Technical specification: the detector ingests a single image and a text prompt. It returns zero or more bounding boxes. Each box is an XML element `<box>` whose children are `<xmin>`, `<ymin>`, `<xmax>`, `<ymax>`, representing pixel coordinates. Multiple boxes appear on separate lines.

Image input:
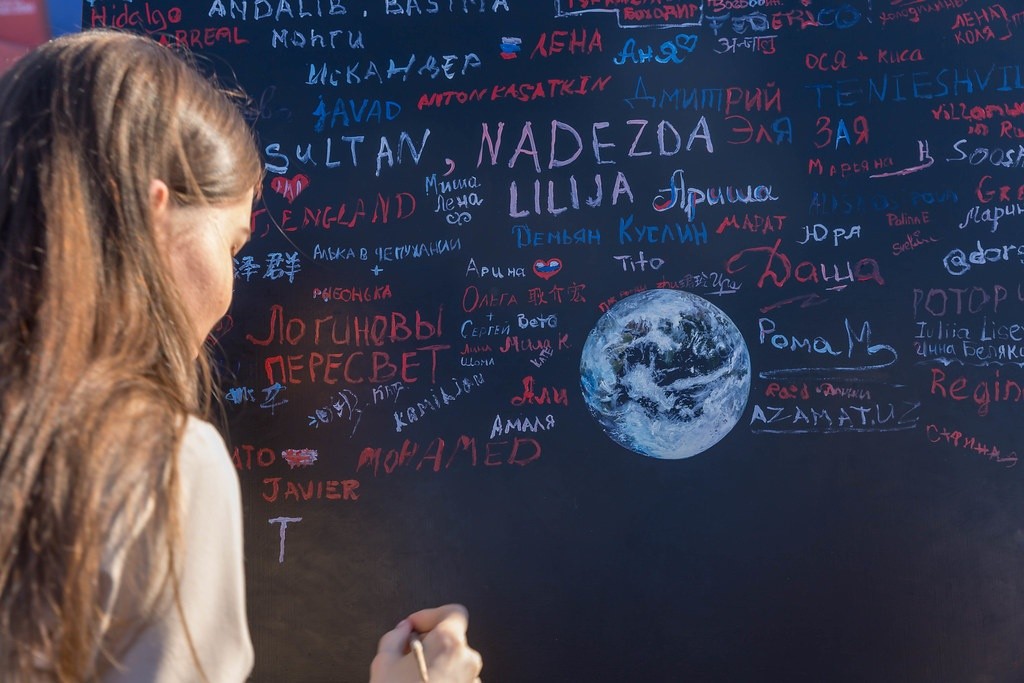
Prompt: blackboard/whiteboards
<box><xmin>82</xmin><ymin>0</ymin><xmax>1024</xmax><ymax>683</ymax></box>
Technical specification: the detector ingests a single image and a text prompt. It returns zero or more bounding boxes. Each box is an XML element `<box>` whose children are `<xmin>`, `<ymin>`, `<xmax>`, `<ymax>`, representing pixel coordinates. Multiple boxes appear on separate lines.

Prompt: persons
<box><xmin>0</xmin><ymin>30</ymin><xmax>483</xmax><ymax>683</ymax></box>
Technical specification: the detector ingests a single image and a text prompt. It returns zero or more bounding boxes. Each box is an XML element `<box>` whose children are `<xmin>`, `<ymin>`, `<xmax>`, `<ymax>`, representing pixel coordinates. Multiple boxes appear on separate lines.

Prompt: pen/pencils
<box><xmin>408</xmin><ymin>632</ymin><xmax>429</xmax><ymax>682</ymax></box>
